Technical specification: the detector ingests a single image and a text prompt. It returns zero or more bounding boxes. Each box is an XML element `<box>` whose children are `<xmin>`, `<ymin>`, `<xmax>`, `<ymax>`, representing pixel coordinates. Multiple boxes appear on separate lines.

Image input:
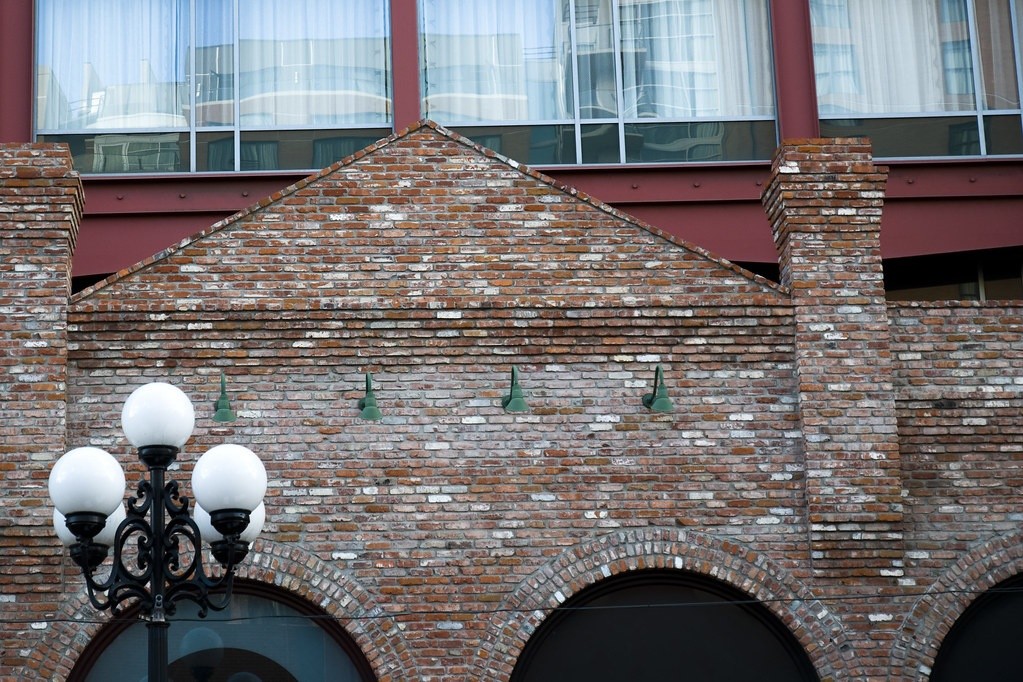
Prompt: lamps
<box><xmin>357</xmin><ymin>372</ymin><xmax>383</xmax><ymax>420</ymax></box>
<box><xmin>501</xmin><ymin>365</ymin><xmax>529</xmax><ymax>414</ymax></box>
<box><xmin>642</xmin><ymin>366</ymin><xmax>675</xmax><ymax>412</ymax></box>
<box><xmin>211</xmin><ymin>373</ymin><xmax>236</xmax><ymax>424</ymax></box>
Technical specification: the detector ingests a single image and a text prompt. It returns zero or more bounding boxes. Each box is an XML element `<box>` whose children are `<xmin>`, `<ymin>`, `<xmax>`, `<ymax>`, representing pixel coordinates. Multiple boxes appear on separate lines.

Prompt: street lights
<box><xmin>43</xmin><ymin>382</ymin><xmax>271</xmax><ymax>682</ymax></box>
